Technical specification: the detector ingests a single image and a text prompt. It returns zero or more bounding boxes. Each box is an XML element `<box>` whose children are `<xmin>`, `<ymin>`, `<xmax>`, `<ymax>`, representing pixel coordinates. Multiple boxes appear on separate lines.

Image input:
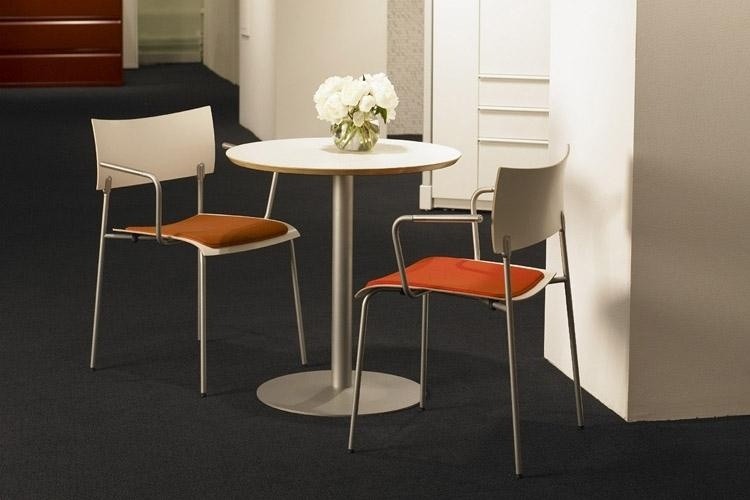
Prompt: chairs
<box><xmin>90</xmin><ymin>105</ymin><xmax>308</xmax><ymax>398</ymax></box>
<box><xmin>348</xmin><ymin>143</ymin><xmax>584</xmax><ymax>477</ymax></box>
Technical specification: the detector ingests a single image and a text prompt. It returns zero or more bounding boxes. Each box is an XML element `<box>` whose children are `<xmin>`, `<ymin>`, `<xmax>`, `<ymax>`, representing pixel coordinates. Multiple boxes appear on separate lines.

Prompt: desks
<box><xmin>226</xmin><ymin>136</ymin><xmax>464</xmax><ymax>418</ymax></box>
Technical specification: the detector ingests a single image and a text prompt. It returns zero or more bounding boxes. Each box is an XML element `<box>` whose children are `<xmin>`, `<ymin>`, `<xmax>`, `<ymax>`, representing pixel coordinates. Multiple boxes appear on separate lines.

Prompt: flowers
<box><xmin>312</xmin><ymin>71</ymin><xmax>401</xmax><ymax>152</ymax></box>
